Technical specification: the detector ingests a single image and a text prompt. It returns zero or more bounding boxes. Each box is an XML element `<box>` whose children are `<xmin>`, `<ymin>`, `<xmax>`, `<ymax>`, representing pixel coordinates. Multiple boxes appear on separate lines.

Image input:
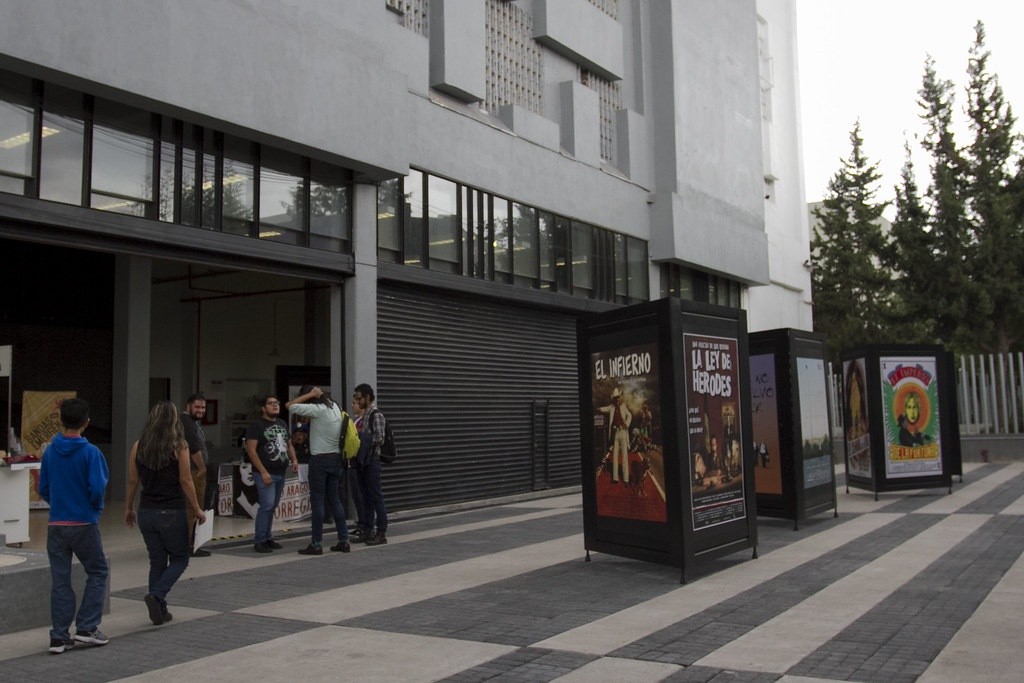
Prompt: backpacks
<box><xmin>368</xmin><ymin>409</ymin><xmax>396</xmax><ymax>466</ymax></box>
<box><xmin>329</xmin><ymin>400</ymin><xmax>361</xmax><ymax>459</ymax></box>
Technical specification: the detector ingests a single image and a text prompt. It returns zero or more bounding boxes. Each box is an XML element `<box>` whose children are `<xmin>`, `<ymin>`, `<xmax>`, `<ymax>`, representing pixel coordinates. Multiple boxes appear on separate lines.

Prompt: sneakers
<box><xmin>49</xmin><ymin>638</ymin><xmax>75</xmax><ymax>653</ymax></box>
<box><xmin>74</xmin><ymin>627</ymin><xmax>109</xmax><ymax>644</ymax></box>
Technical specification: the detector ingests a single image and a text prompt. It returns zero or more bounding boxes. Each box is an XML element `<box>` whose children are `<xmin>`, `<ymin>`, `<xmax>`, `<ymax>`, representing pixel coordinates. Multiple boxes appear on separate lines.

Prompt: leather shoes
<box><xmin>330</xmin><ymin>542</ymin><xmax>350</xmax><ymax>552</ymax></box>
<box><xmin>297</xmin><ymin>544</ymin><xmax>323</xmax><ymax>555</ymax></box>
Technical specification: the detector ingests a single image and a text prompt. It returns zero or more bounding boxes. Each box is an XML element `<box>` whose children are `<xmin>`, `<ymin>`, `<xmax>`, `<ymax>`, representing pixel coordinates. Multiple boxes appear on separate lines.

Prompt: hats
<box><xmin>611</xmin><ymin>388</ymin><xmax>622</xmax><ymax>400</ymax></box>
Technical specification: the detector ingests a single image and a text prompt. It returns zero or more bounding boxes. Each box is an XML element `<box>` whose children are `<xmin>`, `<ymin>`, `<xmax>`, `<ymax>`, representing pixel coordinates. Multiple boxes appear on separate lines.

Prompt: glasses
<box><xmin>265</xmin><ymin>401</ymin><xmax>280</xmax><ymax>405</ymax></box>
<box><xmin>355</xmin><ymin>397</ymin><xmax>363</xmax><ymax>401</ymax></box>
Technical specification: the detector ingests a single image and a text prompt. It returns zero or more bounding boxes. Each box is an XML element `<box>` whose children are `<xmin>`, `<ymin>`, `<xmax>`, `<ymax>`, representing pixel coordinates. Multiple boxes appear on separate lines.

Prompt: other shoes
<box><xmin>190</xmin><ymin>548</ymin><xmax>211</xmax><ymax>557</ymax></box>
<box><xmin>267</xmin><ymin>540</ymin><xmax>282</xmax><ymax>549</ymax></box>
<box><xmin>152</xmin><ymin>612</ymin><xmax>173</xmax><ymax>625</ymax></box>
<box><xmin>350</xmin><ymin>527</ymin><xmax>374</xmax><ymax>543</ymax></box>
<box><xmin>255</xmin><ymin>543</ymin><xmax>273</xmax><ymax>553</ymax></box>
<box><xmin>366</xmin><ymin>528</ymin><xmax>387</xmax><ymax>545</ymax></box>
<box><xmin>144</xmin><ymin>595</ymin><xmax>161</xmax><ymax>622</ymax></box>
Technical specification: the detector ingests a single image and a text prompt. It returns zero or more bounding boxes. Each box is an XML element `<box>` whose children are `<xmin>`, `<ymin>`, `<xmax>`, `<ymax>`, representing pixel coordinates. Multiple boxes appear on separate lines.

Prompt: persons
<box><xmin>906</xmin><ymin>393</ymin><xmax>920</xmax><ymax>423</ymax></box>
<box><xmin>180</xmin><ymin>394</ymin><xmax>211</xmax><ymax>557</ymax></box>
<box><xmin>754</xmin><ymin>440</ymin><xmax>768</xmax><ymax>468</ymax></box>
<box><xmin>124</xmin><ymin>401</ymin><xmax>206</xmax><ymax>625</ymax></box>
<box><xmin>247</xmin><ymin>396</ymin><xmax>298</xmax><ymax>552</ymax></box>
<box><xmin>693</xmin><ymin>399</ymin><xmax>741</xmax><ymax>488</ymax></box>
<box><xmin>596</xmin><ymin>388</ymin><xmax>652</xmax><ymax>487</ymax></box>
<box><xmin>286</xmin><ymin>385</ymin><xmax>350</xmax><ymax>554</ymax></box>
<box><xmin>350</xmin><ymin>383</ymin><xmax>388</xmax><ymax>545</ymax></box>
<box><xmin>38</xmin><ymin>398</ymin><xmax>110</xmax><ymax>653</ymax></box>
